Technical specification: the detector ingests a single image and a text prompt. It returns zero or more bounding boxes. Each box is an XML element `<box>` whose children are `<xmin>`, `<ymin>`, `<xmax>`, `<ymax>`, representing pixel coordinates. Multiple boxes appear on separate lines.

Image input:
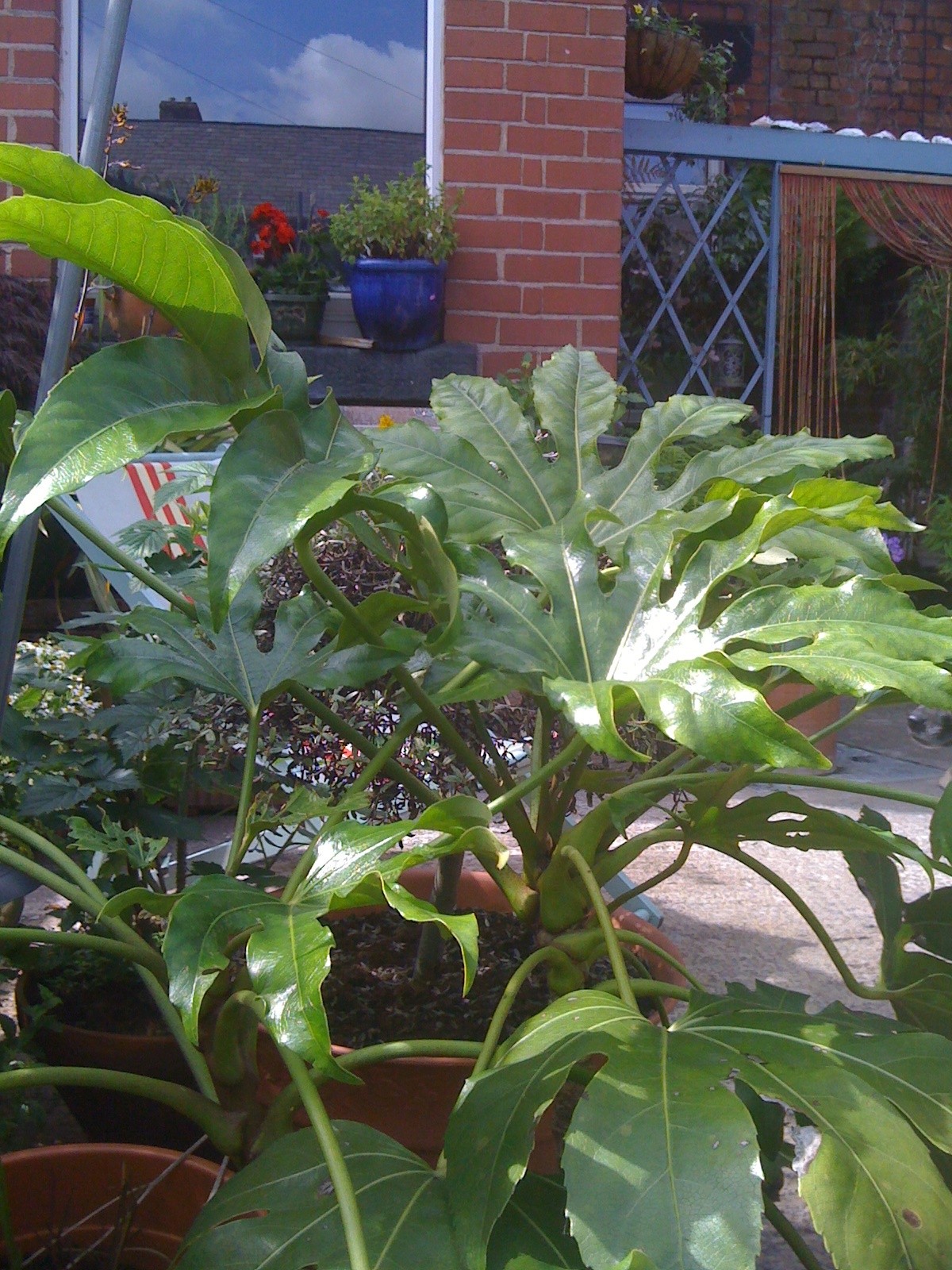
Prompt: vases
<box><xmin>627</xmin><ymin>22</ymin><xmax>700</xmax><ymax>96</ymax></box>
<box><xmin>262</xmin><ymin>295</ymin><xmax>335</xmax><ymax>345</ymax></box>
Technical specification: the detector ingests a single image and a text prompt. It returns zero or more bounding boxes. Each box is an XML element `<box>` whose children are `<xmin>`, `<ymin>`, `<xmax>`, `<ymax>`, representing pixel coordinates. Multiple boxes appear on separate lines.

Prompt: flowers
<box><xmin>244</xmin><ymin>201</ymin><xmax>330</xmax><ymax>292</ymax></box>
<box><xmin>625</xmin><ymin>2</ymin><xmax>746</xmax><ymax>123</ymax></box>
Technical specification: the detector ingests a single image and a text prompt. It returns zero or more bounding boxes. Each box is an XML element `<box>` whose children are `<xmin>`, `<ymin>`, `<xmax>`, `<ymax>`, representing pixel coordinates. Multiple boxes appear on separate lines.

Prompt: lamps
<box><xmin>709</xmin><ymin>331</ymin><xmax>746</xmax><ymax>398</ymax></box>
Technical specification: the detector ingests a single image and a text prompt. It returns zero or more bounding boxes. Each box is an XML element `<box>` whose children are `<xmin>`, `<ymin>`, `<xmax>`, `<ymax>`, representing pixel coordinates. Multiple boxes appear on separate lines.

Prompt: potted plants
<box><xmin>1</xmin><ymin>142</ymin><xmax>952</xmax><ymax>1269</ymax></box>
